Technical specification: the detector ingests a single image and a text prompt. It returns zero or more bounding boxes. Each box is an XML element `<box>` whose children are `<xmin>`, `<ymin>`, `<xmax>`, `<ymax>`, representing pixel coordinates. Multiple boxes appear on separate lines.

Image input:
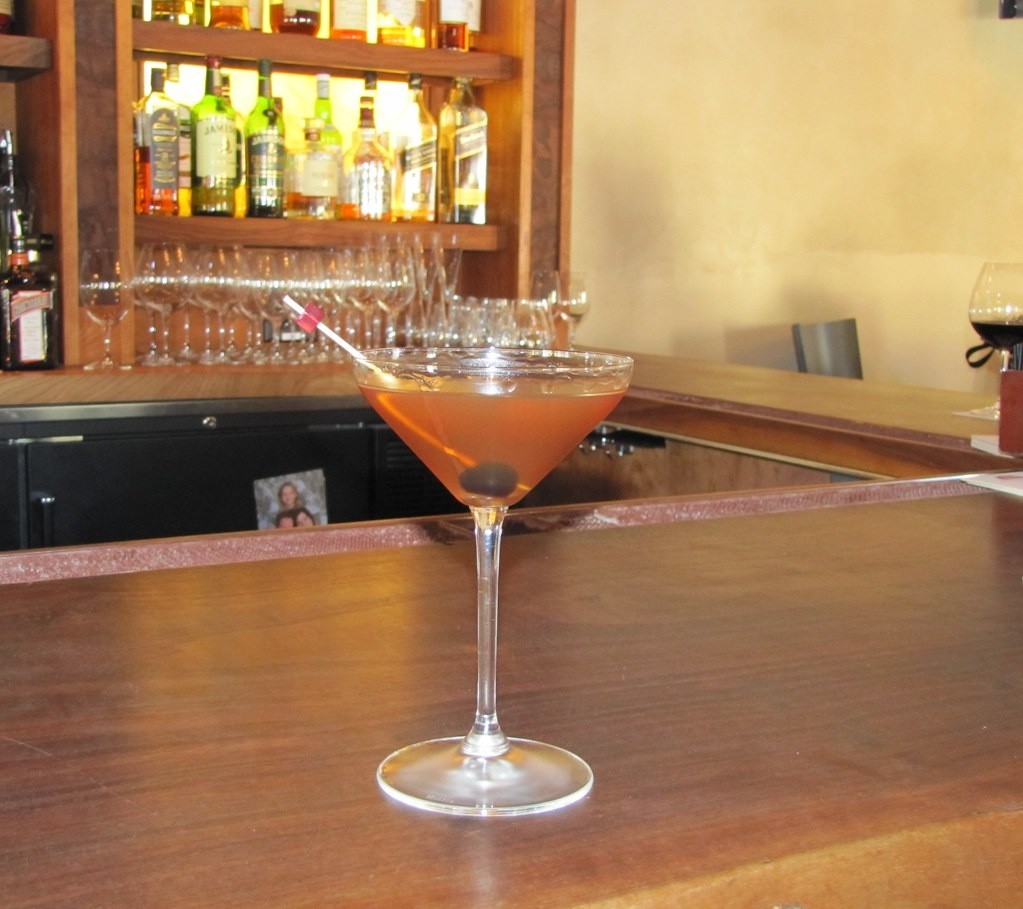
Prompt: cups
<box><xmin>967</xmin><ymin>260</ymin><xmax>1023</xmax><ymax>418</ymax></box>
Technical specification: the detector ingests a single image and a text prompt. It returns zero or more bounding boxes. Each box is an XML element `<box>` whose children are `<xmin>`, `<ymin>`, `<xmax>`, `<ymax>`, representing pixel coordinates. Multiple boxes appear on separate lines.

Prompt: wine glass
<box><xmin>76</xmin><ymin>233</ymin><xmax>591</xmax><ymax>369</ymax></box>
<box><xmin>351</xmin><ymin>347</ymin><xmax>634</xmax><ymax>815</ymax></box>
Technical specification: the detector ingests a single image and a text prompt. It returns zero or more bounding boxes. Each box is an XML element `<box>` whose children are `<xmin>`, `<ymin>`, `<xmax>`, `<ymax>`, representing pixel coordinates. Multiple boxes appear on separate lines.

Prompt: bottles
<box><xmin>132</xmin><ymin>0</ymin><xmax>489</xmax><ymax>225</ymax></box>
<box><xmin>0</xmin><ymin>129</ymin><xmax>61</xmax><ymax>368</ymax></box>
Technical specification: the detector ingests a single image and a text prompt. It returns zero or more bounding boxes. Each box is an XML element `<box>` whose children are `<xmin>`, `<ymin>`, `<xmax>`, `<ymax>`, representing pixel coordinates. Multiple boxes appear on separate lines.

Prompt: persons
<box><xmin>277</xmin><ymin>482</ymin><xmax>305</xmax><ymax>512</ymax></box>
<box><xmin>275</xmin><ymin>506</ymin><xmax>315</xmax><ymax>529</ymax></box>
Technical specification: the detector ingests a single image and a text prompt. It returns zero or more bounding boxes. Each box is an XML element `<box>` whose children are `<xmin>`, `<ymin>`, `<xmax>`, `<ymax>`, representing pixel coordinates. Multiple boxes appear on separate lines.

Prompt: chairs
<box><xmin>791</xmin><ymin>316</ymin><xmax>863</xmax><ymax>381</ymax></box>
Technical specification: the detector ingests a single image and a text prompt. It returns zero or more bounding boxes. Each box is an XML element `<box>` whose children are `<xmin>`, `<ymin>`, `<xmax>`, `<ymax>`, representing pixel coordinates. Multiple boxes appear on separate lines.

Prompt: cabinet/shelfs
<box><xmin>25</xmin><ymin>424</ymin><xmax>375</xmax><ymax>551</ymax></box>
<box><xmin>115</xmin><ymin>0</ymin><xmax>537</xmax><ymax>367</ymax></box>
<box><xmin>0</xmin><ymin>0</ymin><xmax>80</xmax><ymax>370</ymax></box>
